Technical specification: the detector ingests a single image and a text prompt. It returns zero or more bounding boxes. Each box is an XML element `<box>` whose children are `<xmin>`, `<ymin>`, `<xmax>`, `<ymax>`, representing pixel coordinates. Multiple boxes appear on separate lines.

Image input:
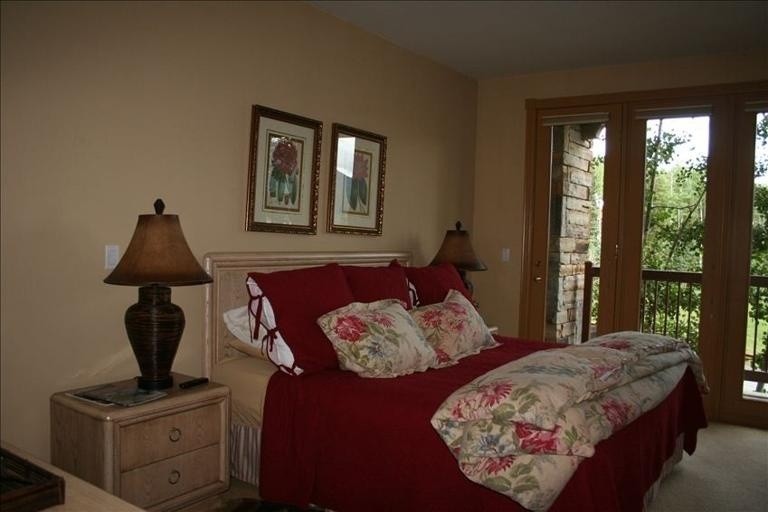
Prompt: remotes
<box><xmin>178</xmin><ymin>378</ymin><xmax>209</xmax><ymax>390</ymax></box>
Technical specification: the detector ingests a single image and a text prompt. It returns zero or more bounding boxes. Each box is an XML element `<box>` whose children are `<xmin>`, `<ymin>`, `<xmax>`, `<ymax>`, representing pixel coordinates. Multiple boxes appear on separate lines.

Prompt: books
<box><xmin>65</xmin><ymin>386</ymin><xmax>115</xmax><ymax>406</ymax></box>
<box><xmin>79</xmin><ymin>385</ymin><xmax>168</xmax><ymax>407</ymax></box>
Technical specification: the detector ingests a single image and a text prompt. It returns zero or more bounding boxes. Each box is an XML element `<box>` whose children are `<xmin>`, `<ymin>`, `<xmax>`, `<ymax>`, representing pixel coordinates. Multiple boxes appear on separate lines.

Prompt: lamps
<box><xmin>103</xmin><ymin>198</ymin><xmax>213</xmax><ymax>391</ymax></box>
<box><xmin>427</xmin><ymin>221</ymin><xmax>487</xmax><ymax>294</ymax></box>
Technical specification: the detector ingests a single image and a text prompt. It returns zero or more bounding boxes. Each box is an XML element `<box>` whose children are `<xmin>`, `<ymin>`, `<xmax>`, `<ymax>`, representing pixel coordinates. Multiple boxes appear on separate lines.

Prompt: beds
<box><xmin>203</xmin><ymin>250</ymin><xmax>710</xmax><ymax>512</ymax></box>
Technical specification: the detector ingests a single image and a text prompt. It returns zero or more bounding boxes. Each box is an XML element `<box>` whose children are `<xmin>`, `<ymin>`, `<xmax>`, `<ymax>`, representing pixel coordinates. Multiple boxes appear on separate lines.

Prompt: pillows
<box><xmin>341</xmin><ymin>259</ymin><xmax>412</xmax><ymax>311</ymax></box>
<box><xmin>316</xmin><ymin>298</ymin><xmax>439</xmax><ymax>379</ymax></box>
<box><xmin>228</xmin><ymin>339</ymin><xmax>263</xmax><ymax>357</ymax></box>
<box><xmin>245</xmin><ymin>259</ymin><xmax>356</xmax><ymax>377</ymax></box>
<box><xmin>403</xmin><ymin>252</ymin><xmax>478</xmax><ymax>311</ymax></box>
<box><xmin>408</xmin><ymin>289</ymin><xmax>504</xmax><ymax>368</ymax></box>
<box><xmin>223</xmin><ymin>303</ymin><xmax>267</xmax><ymax>348</ymax></box>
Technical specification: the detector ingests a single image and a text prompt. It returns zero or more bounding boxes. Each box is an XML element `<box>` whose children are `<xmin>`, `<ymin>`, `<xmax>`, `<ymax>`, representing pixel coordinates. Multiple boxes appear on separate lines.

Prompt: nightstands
<box><xmin>50</xmin><ymin>372</ymin><xmax>232</xmax><ymax>512</ymax></box>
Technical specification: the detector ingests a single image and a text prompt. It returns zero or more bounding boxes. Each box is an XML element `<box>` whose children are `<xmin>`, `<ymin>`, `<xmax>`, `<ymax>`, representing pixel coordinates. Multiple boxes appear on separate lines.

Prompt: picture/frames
<box><xmin>244</xmin><ymin>104</ymin><xmax>323</xmax><ymax>236</ymax></box>
<box><xmin>326</xmin><ymin>122</ymin><xmax>387</xmax><ymax>236</ymax></box>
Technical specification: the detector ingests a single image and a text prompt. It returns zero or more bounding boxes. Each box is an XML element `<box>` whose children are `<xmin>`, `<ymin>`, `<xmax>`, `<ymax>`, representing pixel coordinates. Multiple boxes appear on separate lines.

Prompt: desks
<box><xmin>0</xmin><ymin>440</ymin><xmax>147</xmax><ymax>512</ymax></box>
